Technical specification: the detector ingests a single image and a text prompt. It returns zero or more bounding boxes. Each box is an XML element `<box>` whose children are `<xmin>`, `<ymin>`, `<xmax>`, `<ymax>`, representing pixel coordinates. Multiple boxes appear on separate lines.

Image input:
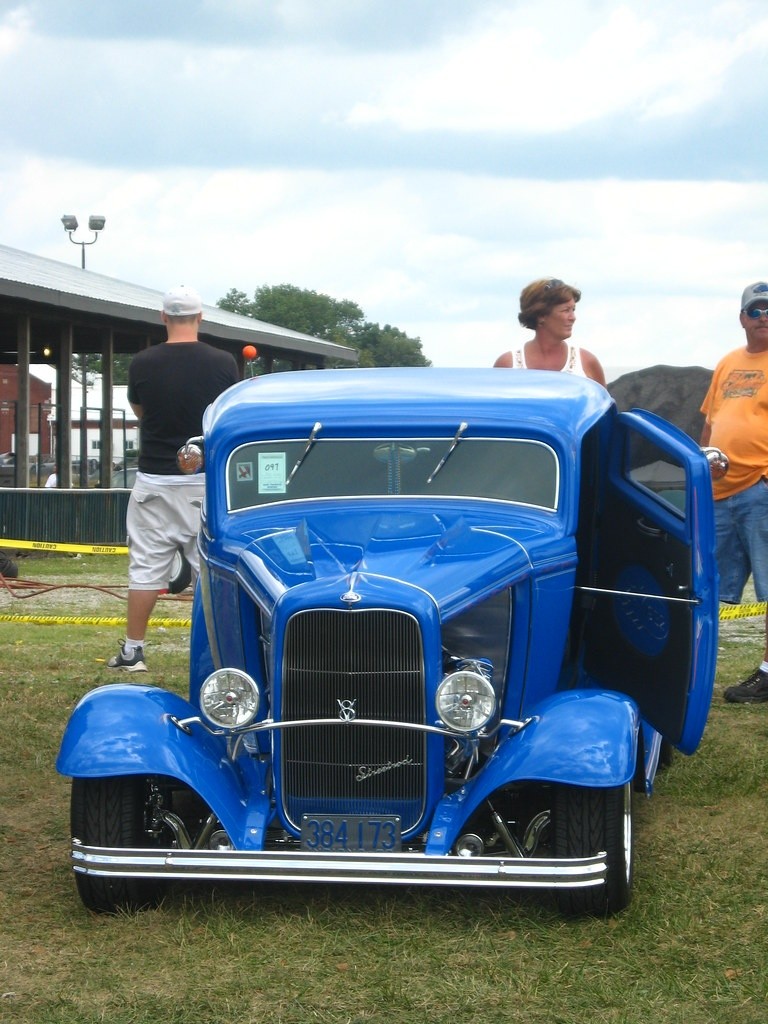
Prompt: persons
<box><xmin>492</xmin><ymin>278</ymin><xmax>607</xmax><ymax>391</ymax></box>
<box><xmin>701</xmin><ymin>282</ymin><xmax>768</xmax><ymax>704</ymax></box>
<box><xmin>107</xmin><ymin>284</ymin><xmax>241</xmax><ymax>671</ymax></box>
<box><xmin>45</xmin><ymin>464</ymin><xmax>74</xmax><ymax>487</ymax></box>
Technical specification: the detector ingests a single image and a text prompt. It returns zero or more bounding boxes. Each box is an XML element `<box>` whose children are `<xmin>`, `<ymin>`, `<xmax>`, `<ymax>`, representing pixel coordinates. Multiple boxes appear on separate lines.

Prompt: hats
<box><xmin>741</xmin><ymin>281</ymin><xmax>768</xmax><ymax>312</ymax></box>
<box><xmin>163</xmin><ymin>285</ymin><xmax>202</xmax><ymax>316</ymax></box>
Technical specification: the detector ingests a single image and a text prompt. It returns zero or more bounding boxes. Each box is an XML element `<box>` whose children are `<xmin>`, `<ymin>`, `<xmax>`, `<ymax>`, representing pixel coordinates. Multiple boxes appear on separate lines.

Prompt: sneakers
<box><xmin>107</xmin><ymin>638</ymin><xmax>147</xmax><ymax>671</ymax></box>
<box><xmin>722</xmin><ymin>667</ymin><xmax>768</xmax><ymax>704</ymax></box>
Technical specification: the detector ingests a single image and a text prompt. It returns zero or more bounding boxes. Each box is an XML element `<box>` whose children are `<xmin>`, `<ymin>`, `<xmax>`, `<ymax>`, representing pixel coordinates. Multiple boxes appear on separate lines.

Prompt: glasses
<box><xmin>545</xmin><ymin>279</ymin><xmax>563</xmax><ymax>292</ymax></box>
<box><xmin>743</xmin><ymin>308</ymin><xmax>768</xmax><ymax>319</ymax></box>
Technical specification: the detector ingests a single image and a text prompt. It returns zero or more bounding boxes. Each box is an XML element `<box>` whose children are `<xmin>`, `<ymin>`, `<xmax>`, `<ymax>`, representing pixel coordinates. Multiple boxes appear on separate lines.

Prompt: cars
<box><xmin>52</xmin><ymin>367</ymin><xmax>721</xmax><ymax>918</ymax></box>
<box><xmin>0</xmin><ymin>452</ymin><xmax>138</xmax><ymax>489</ymax></box>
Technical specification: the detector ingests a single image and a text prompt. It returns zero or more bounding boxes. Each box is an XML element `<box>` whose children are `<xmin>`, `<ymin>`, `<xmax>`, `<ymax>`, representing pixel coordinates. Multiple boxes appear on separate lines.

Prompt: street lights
<box><xmin>60</xmin><ymin>214</ymin><xmax>107</xmax><ymax>268</ymax></box>
